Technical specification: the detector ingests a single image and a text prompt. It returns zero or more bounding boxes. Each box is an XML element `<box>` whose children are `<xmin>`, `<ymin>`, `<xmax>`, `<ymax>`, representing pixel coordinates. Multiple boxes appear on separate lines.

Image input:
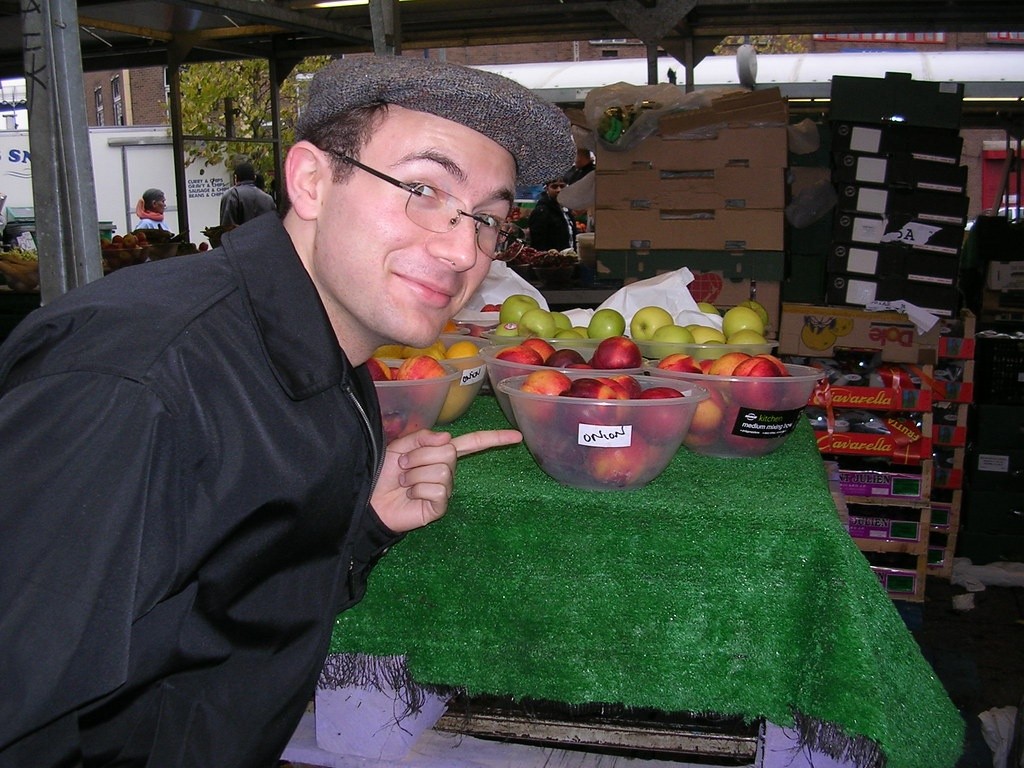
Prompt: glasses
<box><xmin>322</xmin><ymin>147</ymin><xmax>529</xmax><ymax>263</ymax></box>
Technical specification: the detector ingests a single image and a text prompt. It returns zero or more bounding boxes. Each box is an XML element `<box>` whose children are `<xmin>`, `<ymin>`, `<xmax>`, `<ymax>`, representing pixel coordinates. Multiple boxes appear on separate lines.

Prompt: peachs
<box><xmin>652</xmin><ymin>349</ymin><xmax>807</xmax><ymax>456</ymax></box>
<box><xmin>488</xmin><ymin>336</ymin><xmax>691</xmax><ymax>486</ymax></box>
<box><xmin>440</xmin><ymin>303</ymin><xmax>504</xmax><ymax>338</ymax></box>
<box><xmin>199</xmin><ymin>242</ymin><xmax>209</xmax><ymax>251</ymax></box>
<box><xmin>358</xmin><ymin>353</ymin><xmax>449</xmax><ymax>442</ymax></box>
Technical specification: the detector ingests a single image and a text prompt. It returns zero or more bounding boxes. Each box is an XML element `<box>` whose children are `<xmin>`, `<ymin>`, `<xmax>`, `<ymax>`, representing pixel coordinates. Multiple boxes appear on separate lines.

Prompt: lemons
<box><xmin>372</xmin><ymin>336</ymin><xmax>483</xmax><ymax>424</ymax></box>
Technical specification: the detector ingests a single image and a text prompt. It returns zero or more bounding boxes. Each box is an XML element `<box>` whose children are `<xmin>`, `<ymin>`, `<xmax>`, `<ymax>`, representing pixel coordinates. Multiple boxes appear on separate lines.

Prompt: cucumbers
<box><xmin>131</xmin><ymin>223</ymin><xmax>190</xmax><ymax>244</ymax></box>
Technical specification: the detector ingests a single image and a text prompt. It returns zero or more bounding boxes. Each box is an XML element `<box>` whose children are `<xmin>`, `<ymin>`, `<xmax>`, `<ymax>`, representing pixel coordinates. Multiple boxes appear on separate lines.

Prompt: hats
<box><xmin>295</xmin><ymin>56</ymin><xmax>576</xmax><ymax>185</ymax></box>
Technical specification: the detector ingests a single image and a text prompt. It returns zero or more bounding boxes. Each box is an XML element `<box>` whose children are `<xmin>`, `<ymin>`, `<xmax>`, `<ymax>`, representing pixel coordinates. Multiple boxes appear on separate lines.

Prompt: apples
<box><xmin>491</xmin><ymin>295</ymin><xmax>769</xmax><ymax>360</ymax></box>
<box><xmin>99</xmin><ymin>232</ymin><xmax>152</xmax><ymax>270</ymax></box>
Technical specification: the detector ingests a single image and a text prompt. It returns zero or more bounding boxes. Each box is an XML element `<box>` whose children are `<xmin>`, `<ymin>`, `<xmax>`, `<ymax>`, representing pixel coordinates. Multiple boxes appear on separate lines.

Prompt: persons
<box><xmin>135</xmin><ymin>188</ymin><xmax>169</xmax><ymax>230</ymax></box>
<box><xmin>529</xmin><ymin>175</ymin><xmax>577</xmax><ymax>253</ymax></box>
<box><xmin>564</xmin><ymin>148</ymin><xmax>595</xmax><ymax>224</ymax></box>
<box><xmin>220</xmin><ymin>164</ymin><xmax>276</xmax><ymax>232</ymax></box>
<box><xmin>0</xmin><ymin>55</ymin><xmax>578</xmax><ymax>768</ymax></box>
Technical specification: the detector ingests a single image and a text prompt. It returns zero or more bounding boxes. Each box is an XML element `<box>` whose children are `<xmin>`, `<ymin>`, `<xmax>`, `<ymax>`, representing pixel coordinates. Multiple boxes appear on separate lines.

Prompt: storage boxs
<box><xmin>591</xmin><ymin>71</ymin><xmax>1024</xmax><ymax>603</ymax></box>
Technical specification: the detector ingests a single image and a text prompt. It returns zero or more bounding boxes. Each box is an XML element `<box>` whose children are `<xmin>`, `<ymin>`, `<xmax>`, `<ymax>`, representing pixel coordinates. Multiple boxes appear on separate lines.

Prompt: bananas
<box><xmin>0</xmin><ymin>252</ymin><xmax>41</xmax><ymax>291</ymax></box>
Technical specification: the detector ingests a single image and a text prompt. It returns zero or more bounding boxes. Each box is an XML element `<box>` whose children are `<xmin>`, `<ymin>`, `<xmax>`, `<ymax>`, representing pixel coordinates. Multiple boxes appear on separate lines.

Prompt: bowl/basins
<box><xmin>101</xmin><ymin>241</ymin><xmax>181</xmax><ymax>270</ymax></box>
<box><xmin>358</xmin><ymin>308</ymin><xmax>827</xmax><ymax>494</ymax></box>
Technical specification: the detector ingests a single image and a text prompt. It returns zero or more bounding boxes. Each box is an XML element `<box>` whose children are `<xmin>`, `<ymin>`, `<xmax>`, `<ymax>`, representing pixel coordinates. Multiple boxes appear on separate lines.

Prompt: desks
<box><xmin>327</xmin><ymin>395</ymin><xmax>967</xmax><ymax>768</ymax></box>
